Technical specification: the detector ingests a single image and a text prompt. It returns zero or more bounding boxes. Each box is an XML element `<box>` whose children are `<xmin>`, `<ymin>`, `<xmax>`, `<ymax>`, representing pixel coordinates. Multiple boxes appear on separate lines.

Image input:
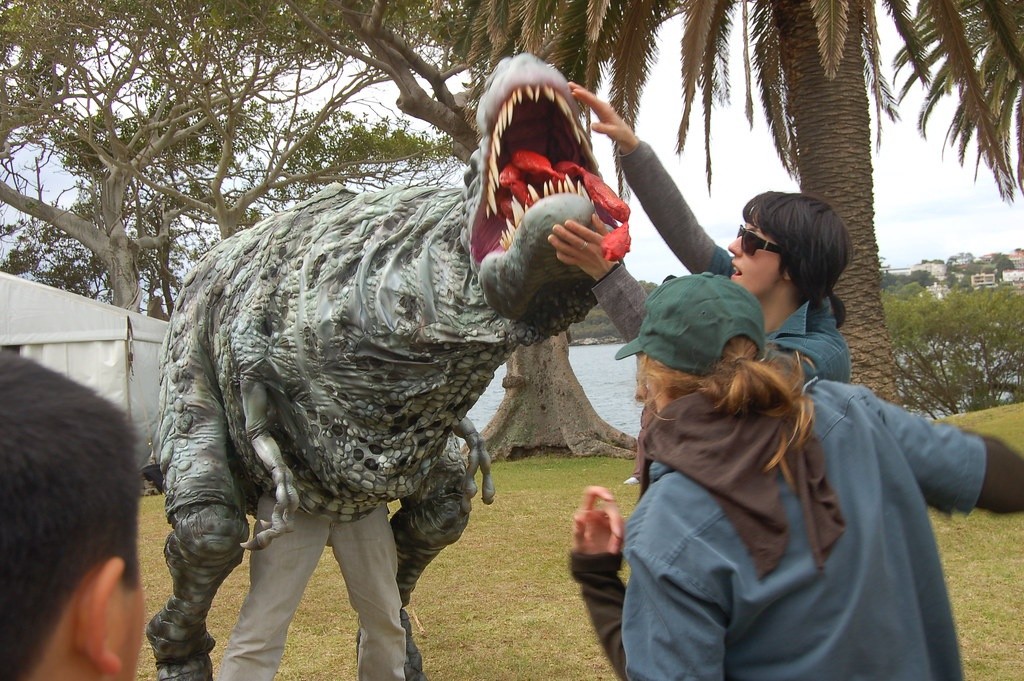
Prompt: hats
<box><xmin>615</xmin><ymin>272</ymin><xmax>767</xmax><ymax>375</ymax></box>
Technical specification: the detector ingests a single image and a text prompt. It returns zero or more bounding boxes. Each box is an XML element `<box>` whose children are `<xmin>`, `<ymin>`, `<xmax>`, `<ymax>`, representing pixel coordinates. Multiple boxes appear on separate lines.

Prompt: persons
<box><xmin>217</xmin><ymin>493</ymin><xmax>407</xmax><ymax>681</ymax></box>
<box><xmin>547</xmin><ymin>81</ymin><xmax>854</xmax><ymax>384</ymax></box>
<box><xmin>0</xmin><ymin>350</ymin><xmax>145</xmax><ymax>681</ymax></box>
<box><xmin>571</xmin><ymin>271</ymin><xmax>1024</xmax><ymax>681</ymax></box>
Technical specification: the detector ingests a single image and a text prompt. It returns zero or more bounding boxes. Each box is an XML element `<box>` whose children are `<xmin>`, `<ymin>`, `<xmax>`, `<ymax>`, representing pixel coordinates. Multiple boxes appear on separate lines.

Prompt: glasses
<box><xmin>737</xmin><ymin>225</ymin><xmax>781</xmax><ymax>259</ymax></box>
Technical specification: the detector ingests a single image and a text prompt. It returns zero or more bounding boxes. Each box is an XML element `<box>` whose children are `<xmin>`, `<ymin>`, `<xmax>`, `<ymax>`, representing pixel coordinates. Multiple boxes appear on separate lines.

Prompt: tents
<box><xmin>0</xmin><ymin>271</ymin><xmax>172</xmax><ymax>467</ymax></box>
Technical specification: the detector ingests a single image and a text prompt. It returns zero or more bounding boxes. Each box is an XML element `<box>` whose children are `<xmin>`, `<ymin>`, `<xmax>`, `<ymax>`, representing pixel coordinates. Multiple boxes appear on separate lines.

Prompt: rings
<box><xmin>581</xmin><ymin>241</ymin><xmax>588</xmax><ymax>250</ymax></box>
